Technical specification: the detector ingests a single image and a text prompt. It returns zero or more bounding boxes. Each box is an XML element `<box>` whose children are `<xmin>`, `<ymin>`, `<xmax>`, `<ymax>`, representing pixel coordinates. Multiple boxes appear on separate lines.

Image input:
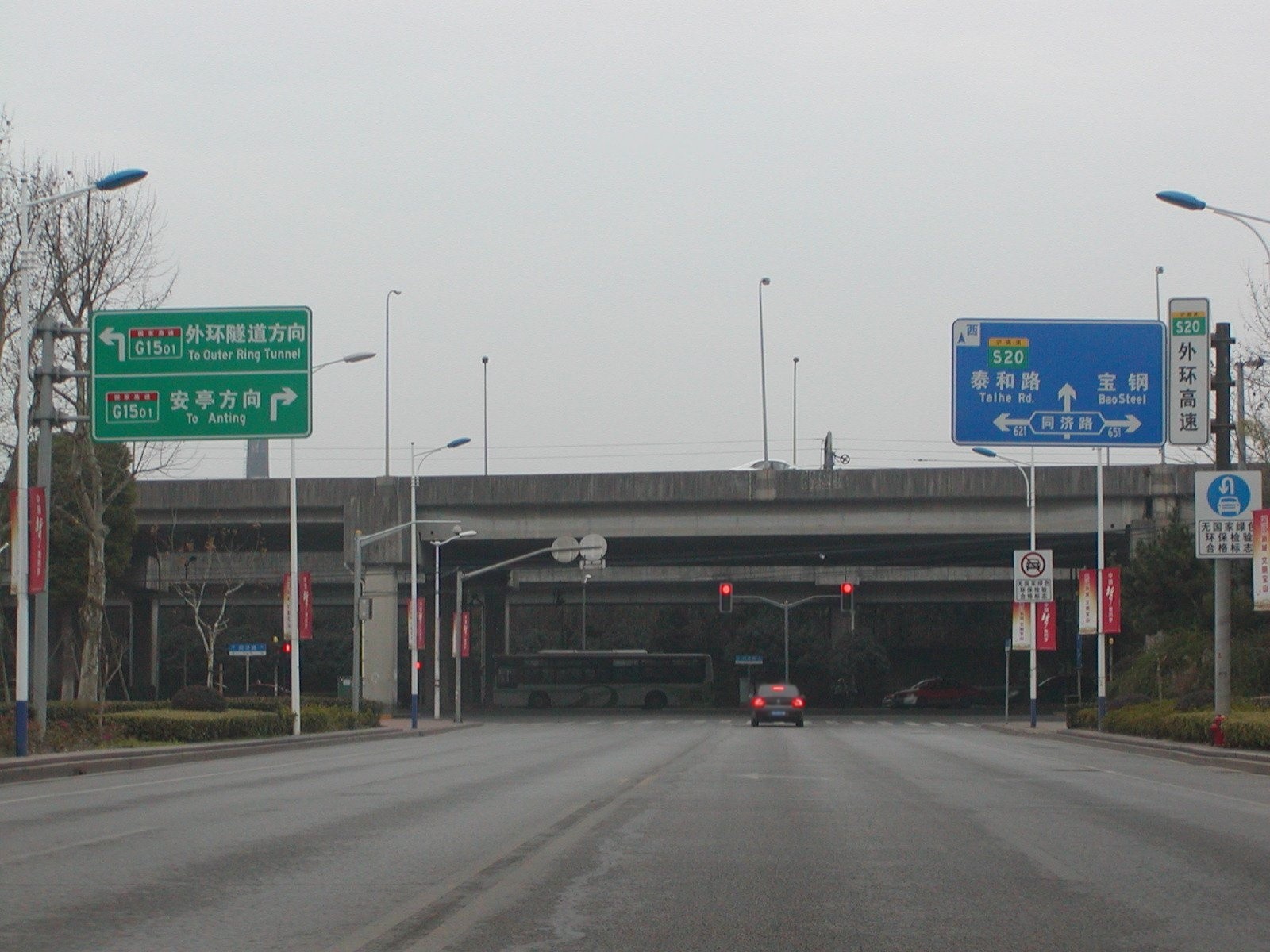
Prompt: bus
<box><xmin>489</xmin><ymin>649</ymin><xmax>715</xmax><ymax>710</ymax></box>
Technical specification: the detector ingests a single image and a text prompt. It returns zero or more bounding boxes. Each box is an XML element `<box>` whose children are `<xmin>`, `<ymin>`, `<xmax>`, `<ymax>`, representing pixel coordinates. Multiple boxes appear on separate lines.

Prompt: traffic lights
<box><xmin>841</xmin><ymin>582</ymin><xmax>852</xmax><ymax>611</ymax></box>
<box><xmin>719</xmin><ymin>581</ymin><xmax>732</xmax><ymax>612</ymax></box>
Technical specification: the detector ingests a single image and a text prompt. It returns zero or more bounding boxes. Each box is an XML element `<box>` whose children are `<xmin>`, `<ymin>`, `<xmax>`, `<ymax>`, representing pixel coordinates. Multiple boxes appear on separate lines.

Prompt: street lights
<box><xmin>481</xmin><ymin>355</ymin><xmax>490</xmax><ymax>477</ymax></box>
<box><xmin>793</xmin><ymin>357</ymin><xmax>800</xmax><ymax>466</ymax></box>
<box><xmin>970</xmin><ymin>447</ymin><xmax>1036</xmax><ymax>728</ymax></box>
<box><xmin>759</xmin><ymin>277</ymin><xmax>771</xmax><ymax>469</ymax></box>
<box><xmin>409</xmin><ymin>437</ymin><xmax>472</xmax><ymax>730</ymax></box>
<box><xmin>1155</xmin><ymin>266</ymin><xmax>1164</xmax><ymax>321</ymax></box>
<box><xmin>385</xmin><ymin>290</ymin><xmax>402</xmax><ymax>477</ymax></box>
<box><xmin>289</xmin><ymin>352</ymin><xmax>379</xmax><ymax>736</ymax></box>
<box><xmin>15</xmin><ymin>167</ymin><xmax>149</xmax><ymax>759</ymax></box>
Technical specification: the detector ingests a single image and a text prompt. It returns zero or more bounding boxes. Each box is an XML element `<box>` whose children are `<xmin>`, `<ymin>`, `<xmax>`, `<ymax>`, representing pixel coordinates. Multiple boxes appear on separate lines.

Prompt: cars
<box><xmin>882</xmin><ymin>677</ymin><xmax>985</xmax><ymax>711</ymax></box>
<box><xmin>728</xmin><ymin>458</ymin><xmax>801</xmax><ymax>470</ymax></box>
<box><xmin>1008</xmin><ymin>674</ymin><xmax>1098</xmax><ymax>707</ymax></box>
<box><xmin>746</xmin><ymin>683</ymin><xmax>807</xmax><ymax>728</ymax></box>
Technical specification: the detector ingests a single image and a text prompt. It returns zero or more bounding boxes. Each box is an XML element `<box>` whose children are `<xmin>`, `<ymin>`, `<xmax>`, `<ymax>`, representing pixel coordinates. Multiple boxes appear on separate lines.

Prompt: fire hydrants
<box><xmin>1209</xmin><ymin>712</ymin><xmax>1225</xmax><ymax>747</ymax></box>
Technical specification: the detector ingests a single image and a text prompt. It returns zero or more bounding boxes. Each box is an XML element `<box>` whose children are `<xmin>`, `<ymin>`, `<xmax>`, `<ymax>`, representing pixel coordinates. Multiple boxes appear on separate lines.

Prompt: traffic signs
<box><xmin>90</xmin><ymin>372</ymin><xmax>314</xmax><ymax>444</ymax></box>
<box><xmin>950</xmin><ymin>317</ymin><xmax>1168</xmax><ymax>449</ymax></box>
<box><xmin>88</xmin><ymin>305</ymin><xmax>312</xmax><ymax>377</ymax></box>
